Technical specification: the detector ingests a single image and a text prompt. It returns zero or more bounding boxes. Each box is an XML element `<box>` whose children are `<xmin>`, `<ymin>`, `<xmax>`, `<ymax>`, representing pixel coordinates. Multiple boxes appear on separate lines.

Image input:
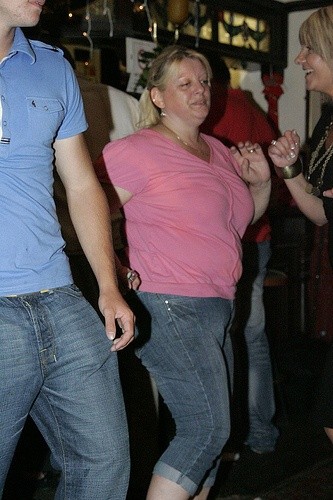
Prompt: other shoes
<box><xmin>151</xmin><ymin>411</ymin><xmax>169</xmax><ymax>429</ymax></box>
<box><xmin>240</xmin><ymin>423</ymin><xmax>280</xmax><ymax>466</ymax></box>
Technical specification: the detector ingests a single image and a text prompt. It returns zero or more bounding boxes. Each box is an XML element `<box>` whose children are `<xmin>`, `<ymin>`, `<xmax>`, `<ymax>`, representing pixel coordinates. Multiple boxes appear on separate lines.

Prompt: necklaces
<box><xmin>176</xmin><ymin>135</ymin><xmax>188</xmax><ymax>146</ymax></box>
<box><xmin>305</xmin><ymin>121</ymin><xmax>333</xmax><ymax>196</ymax></box>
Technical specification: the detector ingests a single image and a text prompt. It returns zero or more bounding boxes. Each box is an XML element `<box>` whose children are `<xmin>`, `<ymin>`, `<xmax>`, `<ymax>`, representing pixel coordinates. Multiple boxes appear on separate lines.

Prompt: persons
<box><xmin>35</xmin><ymin>82</ymin><xmax>139</xmax><ymax>486</ymax></box>
<box><xmin>92</xmin><ymin>44</ymin><xmax>280</xmax><ymax>500</ymax></box>
<box><xmin>0</xmin><ymin>0</ymin><xmax>136</xmax><ymax>500</ymax></box>
<box><xmin>267</xmin><ymin>6</ymin><xmax>333</xmax><ymax>441</ymax></box>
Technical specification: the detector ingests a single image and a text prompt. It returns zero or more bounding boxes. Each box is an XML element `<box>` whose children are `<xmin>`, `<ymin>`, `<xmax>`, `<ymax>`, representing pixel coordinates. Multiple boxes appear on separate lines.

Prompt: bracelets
<box><xmin>274</xmin><ymin>156</ymin><xmax>302</xmax><ymax>180</ymax></box>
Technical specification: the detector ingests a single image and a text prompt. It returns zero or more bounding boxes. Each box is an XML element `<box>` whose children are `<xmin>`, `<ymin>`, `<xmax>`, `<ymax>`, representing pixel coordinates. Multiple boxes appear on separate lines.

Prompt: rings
<box><xmin>249</xmin><ymin>149</ymin><xmax>254</xmax><ymax>153</ymax></box>
<box><xmin>271</xmin><ymin>140</ymin><xmax>276</xmax><ymax>145</ymax></box>
